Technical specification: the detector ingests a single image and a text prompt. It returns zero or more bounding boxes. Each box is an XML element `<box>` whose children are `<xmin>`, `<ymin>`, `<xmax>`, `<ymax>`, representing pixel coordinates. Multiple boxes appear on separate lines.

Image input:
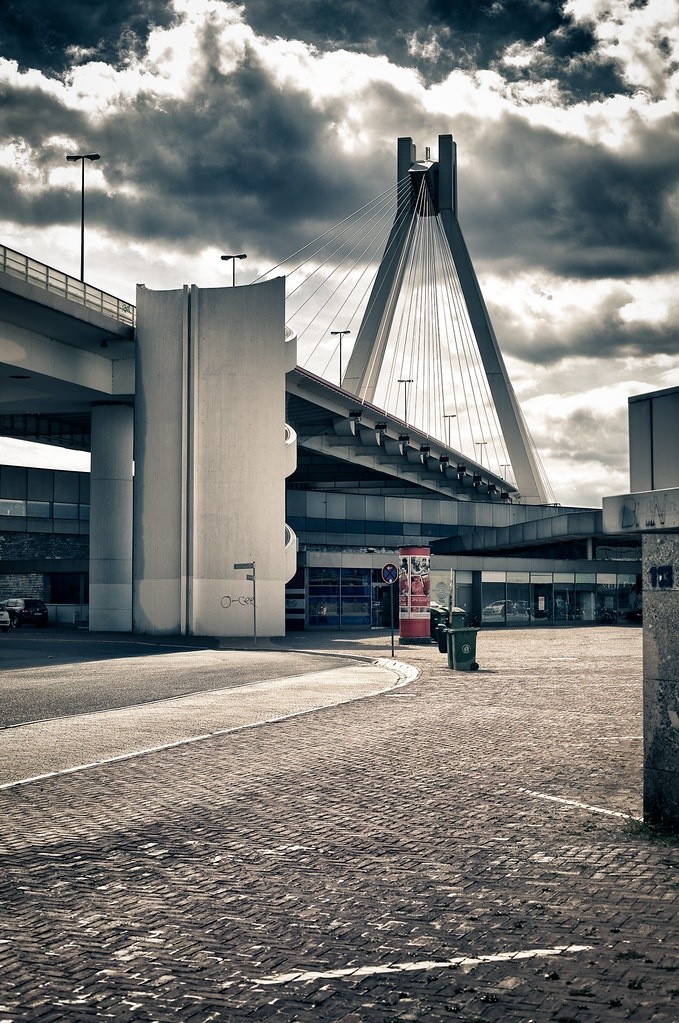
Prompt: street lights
<box><xmin>499</xmin><ymin>465</ymin><xmax>511</xmax><ymax>480</ymax></box>
<box><xmin>63</xmin><ymin>152</ymin><xmax>102</xmax><ymax>283</ymax></box>
<box><xmin>398</xmin><ymin>379</ymin><xmax>414</xmax><ymax>423</ymax></box>
<box><xmin>475</xmin><ymin>442</ymin><xmax>488</xmax><ymax>465</ymax></box>
<box><xmin>220</xmin><ymin>253</ymin><xmax>248</xmax><ymax>286</ymax></box>
<box><xmin>442</xmin><ymin>413</ymin><xmax>457</xmax><ymax>447</ymax></box>
<box><xmin>330</xmin><ymin>330</ymin><xmax>351</xmax><ymax>388</ymax></box>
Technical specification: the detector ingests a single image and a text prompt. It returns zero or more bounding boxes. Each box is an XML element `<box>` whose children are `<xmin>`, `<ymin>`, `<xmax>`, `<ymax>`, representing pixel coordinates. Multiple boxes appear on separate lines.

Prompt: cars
<box><xmin>486</xmin><ymin>599</ymin><xmax>531</xmax><ymax>617</ymax></box>
<box><xmin>0</xmin><ymin>603</ymin><xmax>11</xmax><ymax>632</ymax></box>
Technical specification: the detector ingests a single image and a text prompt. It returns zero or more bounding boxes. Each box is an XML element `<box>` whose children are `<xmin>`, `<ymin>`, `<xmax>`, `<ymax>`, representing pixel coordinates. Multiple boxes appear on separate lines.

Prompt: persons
<box><xmin>398</xmin><ymin>558</ymin><xmax>408</xmax><ymax>573</ymax></box>
<box><xmin>421</xmin><ymin>559</ymin><xmax>430</xmax><ymax>571</ymax></box>
<box><xmin>399</xmin><ymin>574</ymin><xmax>409</xmax><ymax>594</ymax></box>
<box><xmin>423</xmin><ymin>574</ymin><xmax>430</xmax><ymax>597</ymax></box>
<box><xmin>412</xmin><ymin>576</ymin><xmax>424</xmax><ymax>595</ymax></box>
<box><xmin>411</xmin><ymin>557</ymin><xmax>420</xmax><ymax>574</ymax></box>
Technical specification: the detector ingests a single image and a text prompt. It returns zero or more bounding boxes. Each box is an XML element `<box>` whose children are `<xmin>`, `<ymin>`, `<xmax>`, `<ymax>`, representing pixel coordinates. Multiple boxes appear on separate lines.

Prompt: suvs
<box><xmin>0</xmin><ymin>598</ymin><xmax>50</xmax><ymax>629</ymax></box>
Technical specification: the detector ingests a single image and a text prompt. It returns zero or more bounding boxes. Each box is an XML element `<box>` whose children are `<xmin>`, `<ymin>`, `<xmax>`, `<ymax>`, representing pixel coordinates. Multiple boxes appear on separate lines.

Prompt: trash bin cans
<box><xmin>430</xmin><ymin>606</ymin><xmax>466</xmax><ymax>638</ymax></box>
<box><xmin>434</xmin><ymin>627</ymin><xmax>447</xmax><ymax>653</ymax></box>
<box><xmin>443</xmin><ymin>627</ymin><xmax>480</xmax><ymax>670</ymax></box>
